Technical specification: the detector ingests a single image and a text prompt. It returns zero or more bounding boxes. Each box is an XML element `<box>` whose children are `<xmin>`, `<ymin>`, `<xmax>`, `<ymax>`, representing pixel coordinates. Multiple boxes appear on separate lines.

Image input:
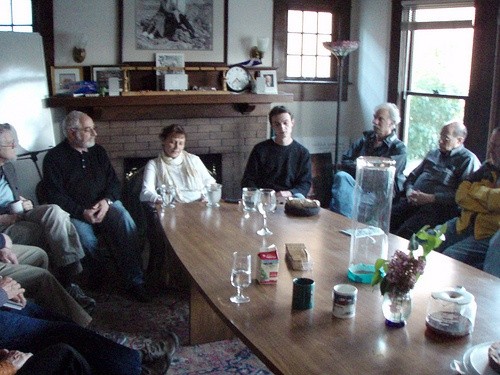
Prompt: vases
<box><xmin>382</xmin><ymin>291</ymin><xmax>416</xmax><ymax>327</ymax></box>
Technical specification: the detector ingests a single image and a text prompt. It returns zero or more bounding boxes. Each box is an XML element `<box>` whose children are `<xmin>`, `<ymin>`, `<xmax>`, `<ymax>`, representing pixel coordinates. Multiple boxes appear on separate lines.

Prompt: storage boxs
<box><xmin>285</xmin><ymin>242</ymin><xmax>313</xmax><ymax>270</ymax></box>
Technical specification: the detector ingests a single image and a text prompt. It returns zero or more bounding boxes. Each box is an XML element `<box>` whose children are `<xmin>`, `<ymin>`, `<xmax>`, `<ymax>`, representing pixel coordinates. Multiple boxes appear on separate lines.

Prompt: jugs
<box><xmin>426</xmin><ymin>286</ymin><xmax>477</xmax><ymax>337</ymax></box>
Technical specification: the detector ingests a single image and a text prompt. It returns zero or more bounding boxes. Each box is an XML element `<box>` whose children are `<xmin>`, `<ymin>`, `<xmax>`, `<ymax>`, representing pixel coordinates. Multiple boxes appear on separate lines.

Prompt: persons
<box><xmin>0</xmin><ymin>233</ymin><xmax>127</xmax><ymax>345</ymax></box>
<box><xmin>160</xmin><ymin>0</ymin><xmax>200</xmax><ymax>41</ymax></box>
<box><xmin>0</xmin><ymin>276</ymin><xmax>178</xmax><ymax>375</ymax></box>
<box><xmin>240</xmin><ymin>106</ymin><xmax>312</xmax><ymax>204</ymax></box>
<box><xmin>140</xmin><ymin>123</ymin><xmax>223</xmax><ymax>299</ymax></box>
<box><xmin>378</xmin><ymin>123</ymin><xmax>483</xmax><ymax>241</ymax></box>
<box><xmin>328</xmin><ymin>103</ymin><xmax>406</xmax><ymax>224</ymax></box>
<box><xmin>442</xmin><ymin>127</ymin><xmax>500</xmax><ymax>278</ymax></box>
<box><xmin>0</xmin><ymin>123</ymin><xmax>96</xmax><ymax>314</ymax></box>
<box><xmin>42</xmin><ymin>111</ymin><xmax>152</xmax><ymax>302</ymax></box>
<box><xmin>0</xmin><ymin>343</ymin><xmax>91</xmax><ymax>375</ymax></box>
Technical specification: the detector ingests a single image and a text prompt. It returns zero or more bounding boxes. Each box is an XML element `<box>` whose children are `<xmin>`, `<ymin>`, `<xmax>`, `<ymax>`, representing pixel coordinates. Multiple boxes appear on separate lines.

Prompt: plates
<box><xmin>463</xmin><ymin>343</ymin><xmax>500</xmax><ymax>375</ymax></box>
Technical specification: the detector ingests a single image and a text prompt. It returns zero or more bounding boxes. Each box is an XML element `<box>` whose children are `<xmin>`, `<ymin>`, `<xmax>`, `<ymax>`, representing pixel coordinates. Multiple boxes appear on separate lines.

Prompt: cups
<box><xmin>346</xmin><ymin>157</ymin><xmax>397</xmax><ymax>284</ymax></box>
<box><xmin>292</xmin><ymin>278</ymin><xmax>315</xmax><ymax>310</ymax></box>
<box><xmin>206</xmin><ymin>184</ymin><xmax>222</xmax><ymax>207</ymax></box>
<box><xmin>8</xmin><ymin>200</ymin><xmax>23</xmax><ymax>215</ymax></box>
<box><xmin>160</xmin><ymin>186</ymin><xmax>175</xmax><ymax>208</ymax></box>
<box><xmin>332</xmin><ymin>284</ymin><xmax>358</xmax><ymax>318</ymax></box>
<box><xmin>243</xmin><ymin>187</ymin><xmax>257</xmax><ymax>211</ymax></box>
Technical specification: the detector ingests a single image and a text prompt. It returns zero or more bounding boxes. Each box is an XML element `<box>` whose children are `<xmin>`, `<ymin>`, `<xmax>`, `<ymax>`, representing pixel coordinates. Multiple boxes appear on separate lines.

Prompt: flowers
<box><xmin>371</xmin><ymin>223</ymin><xmax>447</xmax><ymax>310</ymax></box>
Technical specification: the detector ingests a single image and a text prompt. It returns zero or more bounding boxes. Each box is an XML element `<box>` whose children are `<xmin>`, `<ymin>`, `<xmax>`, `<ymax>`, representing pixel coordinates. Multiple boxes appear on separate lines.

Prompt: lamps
<box><xmin>72</xmin><ymin>30</ymin><xmax>87</xmax><ymax>63</ymax></box>
<box><xmin>119</xmin><ymin>0</ymin><xmax>228</xmax><ymax>67</ymax></box>
<box><xmin>250</xmin><ymin>34</ymin><xmax>270</xmax><ymax>62</ymax></box>
<box><xmin>323</xmin><ymin>40</ymin><xmax>359</xmax><ymax>174</ymax></box>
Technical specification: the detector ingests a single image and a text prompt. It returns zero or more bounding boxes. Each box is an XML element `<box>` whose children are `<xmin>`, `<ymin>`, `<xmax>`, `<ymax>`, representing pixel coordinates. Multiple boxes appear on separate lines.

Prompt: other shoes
<box><xmin>99</xmin><ymin>329</ymin><xmax>129</xmax><ymax>346</ymax></box>
<box><xmin>127</xmin><ymin>283</ymin><xmax>150</xmax><ymax>302</ymax></box>
<box><xmin>146</xmin><ymin>270</ymin><xmax>161</xmax><ymax>295</ymax></box>
<box><xmin>66</xmin><ymin>283</ymin><xmax>96</xmax><ymax>311</ymax></box>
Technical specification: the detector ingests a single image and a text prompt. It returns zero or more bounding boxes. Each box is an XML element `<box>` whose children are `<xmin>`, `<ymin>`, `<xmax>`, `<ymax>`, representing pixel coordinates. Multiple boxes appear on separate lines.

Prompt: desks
<box><xmin>146</xmin><ymin>199</ymin><xmax>500</xmax><ymax>375</ymax></box>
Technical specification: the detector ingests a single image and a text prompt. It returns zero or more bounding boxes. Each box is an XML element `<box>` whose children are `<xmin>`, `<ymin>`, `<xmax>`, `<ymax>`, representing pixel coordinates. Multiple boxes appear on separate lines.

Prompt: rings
<box><xmin>414</xmin><ymin>198</ymin><xmax>416</xmax><ymax>203</ymax></box>
<box><xmin>415</xmin><ymin>203</ymin><xmax>417</xmax><ymax>207</ymax></box>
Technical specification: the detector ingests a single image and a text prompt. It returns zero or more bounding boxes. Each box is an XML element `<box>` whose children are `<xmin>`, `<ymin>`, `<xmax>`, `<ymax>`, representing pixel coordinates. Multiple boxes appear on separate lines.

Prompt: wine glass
<box><xmin>257</xmin><ymin>189</ymin><xmax>276</xmax><ymax>235</ymax></box>
<box><xmin>230</xmin><ymin>252</ymin><xmax>252</xmax><ymax>303</ymax></box>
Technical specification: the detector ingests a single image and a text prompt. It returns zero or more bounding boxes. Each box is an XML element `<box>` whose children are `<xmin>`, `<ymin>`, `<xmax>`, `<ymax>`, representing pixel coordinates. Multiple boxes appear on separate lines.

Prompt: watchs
<box><xmin>104</xmin><ymin>197</ymin><xmax>113</xmax><ymax>206</ymax></box>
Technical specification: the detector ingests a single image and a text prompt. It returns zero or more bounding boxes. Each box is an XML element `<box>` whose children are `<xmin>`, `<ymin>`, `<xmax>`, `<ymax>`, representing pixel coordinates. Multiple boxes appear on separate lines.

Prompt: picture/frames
<box><xmin>225</xmin><ymin>64</ymin><xmax>249</xmax><ymax>92</ymax></box>
<box><xmin>91</xmin><ymin>64</ymin><xmax>130</xmax><ymax>93</ymax></box>
<box><xmin>50</xmin><ymin>66</ymin><xmax>84</xmax><ymax>97</ymax></box>
<box><xmin>257</xmin><ymin>69</ymin><xmax>278</xmax><ymax>95</ymax></box>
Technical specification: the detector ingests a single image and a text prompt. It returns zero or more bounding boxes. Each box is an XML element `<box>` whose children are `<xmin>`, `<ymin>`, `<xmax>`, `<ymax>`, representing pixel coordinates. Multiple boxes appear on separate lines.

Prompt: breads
<box><xmin>288</xmin><ymin>198</ymin><xmax>320</xmax><ymax>207</ymax></box>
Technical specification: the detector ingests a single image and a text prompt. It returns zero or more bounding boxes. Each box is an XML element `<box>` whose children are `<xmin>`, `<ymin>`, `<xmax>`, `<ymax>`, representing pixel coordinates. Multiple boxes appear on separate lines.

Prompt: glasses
<box><xmin>73</xmin><ymin>126</ymin><xmax>97</xmax><ymax>132</ymax></box>
<box><xmin>1</xmin><ymin>143</ymin><xmax>16</xmax><ymax>149</ymax></box>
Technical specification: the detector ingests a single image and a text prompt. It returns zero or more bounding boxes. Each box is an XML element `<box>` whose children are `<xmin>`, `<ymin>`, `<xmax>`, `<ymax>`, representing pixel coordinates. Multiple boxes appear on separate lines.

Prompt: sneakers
<box><xmin>140</xmin><ymin>331</ymin><xmax>179</xmax><ymax>375</ymax></box>
